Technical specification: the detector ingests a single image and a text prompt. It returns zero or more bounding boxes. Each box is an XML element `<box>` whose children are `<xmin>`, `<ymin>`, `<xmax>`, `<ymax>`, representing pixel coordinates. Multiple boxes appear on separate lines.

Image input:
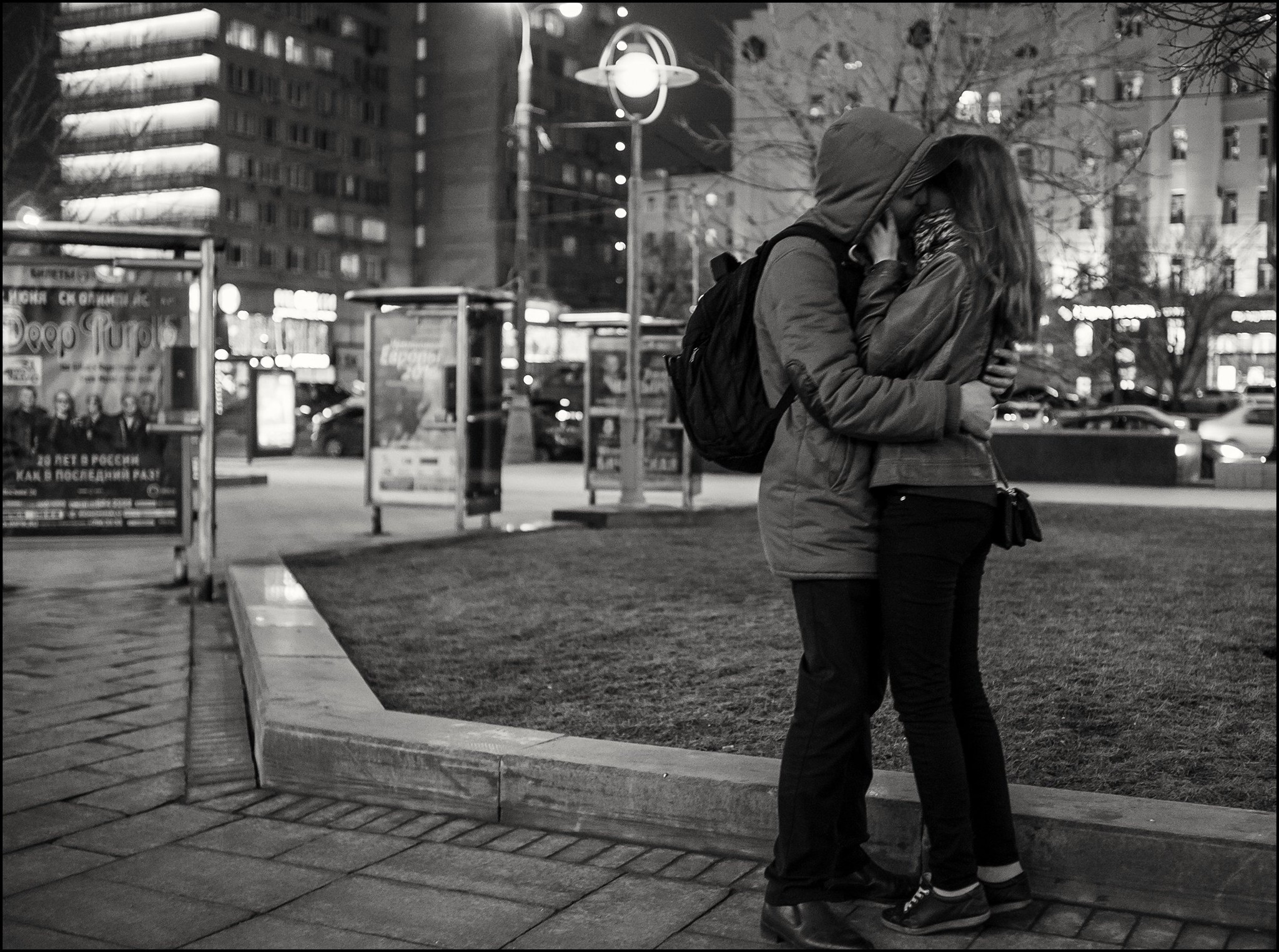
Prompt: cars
<box><xmin>987</xmin><ymin>384</ymin><xmax>1276</xmax><ymax>463</ymax></box>
<box><xmin>214</xmin><ymin>364</ymin><xmax>586</xmax><ymax>465</ymax></box>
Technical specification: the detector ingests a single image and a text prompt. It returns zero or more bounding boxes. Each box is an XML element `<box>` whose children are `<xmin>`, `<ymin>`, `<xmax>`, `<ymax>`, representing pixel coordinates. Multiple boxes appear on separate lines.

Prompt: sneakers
<box><xmin>978</xmin><ymin>871</ymin><xmax>1033</xmax><ymax>914</ymax></box>
<box><xmin>880</xmin><ymin>872</ymin><xmax>991</xmax><ymax>936</ymax></box>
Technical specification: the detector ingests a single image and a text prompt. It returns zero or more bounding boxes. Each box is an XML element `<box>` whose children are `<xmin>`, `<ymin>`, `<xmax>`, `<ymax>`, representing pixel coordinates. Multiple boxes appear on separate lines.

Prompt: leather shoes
<box><xmin>759</xmin><ymin>898</ymin><xmax>874</xmax><ymax>952</ymax></box>
<box><xmin>823</xmin><ymin>848</ymin><xmax>915</xmax><ymax>903</ymax></box>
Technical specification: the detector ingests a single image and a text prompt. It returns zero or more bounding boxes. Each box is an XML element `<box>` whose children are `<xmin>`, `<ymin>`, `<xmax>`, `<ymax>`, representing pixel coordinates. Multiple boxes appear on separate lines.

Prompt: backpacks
<box><xmin>663</xmin><ymin>222</ymin><xmax>857</xmax><ymax>476</ymax></box>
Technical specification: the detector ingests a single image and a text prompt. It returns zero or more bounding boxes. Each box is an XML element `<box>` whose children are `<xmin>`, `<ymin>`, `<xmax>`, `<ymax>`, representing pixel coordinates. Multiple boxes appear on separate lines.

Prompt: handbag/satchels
<box><xmin>992</xmin><ymin>487</ymin><xmax>1042</xmax><ymax>550</ymax></box>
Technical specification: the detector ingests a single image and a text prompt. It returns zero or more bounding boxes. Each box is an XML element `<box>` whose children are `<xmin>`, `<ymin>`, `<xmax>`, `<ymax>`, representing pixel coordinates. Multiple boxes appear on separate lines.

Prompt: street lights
<box><xmin>573</xmin><ymin>22</ymin><xmax>700</xmax><ymax>513</ymax></box>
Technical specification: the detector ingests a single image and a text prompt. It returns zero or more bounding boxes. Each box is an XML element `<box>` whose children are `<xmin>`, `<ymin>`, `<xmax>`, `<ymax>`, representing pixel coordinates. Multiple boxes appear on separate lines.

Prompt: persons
<box><xmin>2</xmin><ymin>385</ymin><xmax>157</xmax><ymax>455</ymax></box>
<box><xmin>751</xmin><ymin>108</ymin><xmax>1045</xmax><ymax>952</ymax></box>
